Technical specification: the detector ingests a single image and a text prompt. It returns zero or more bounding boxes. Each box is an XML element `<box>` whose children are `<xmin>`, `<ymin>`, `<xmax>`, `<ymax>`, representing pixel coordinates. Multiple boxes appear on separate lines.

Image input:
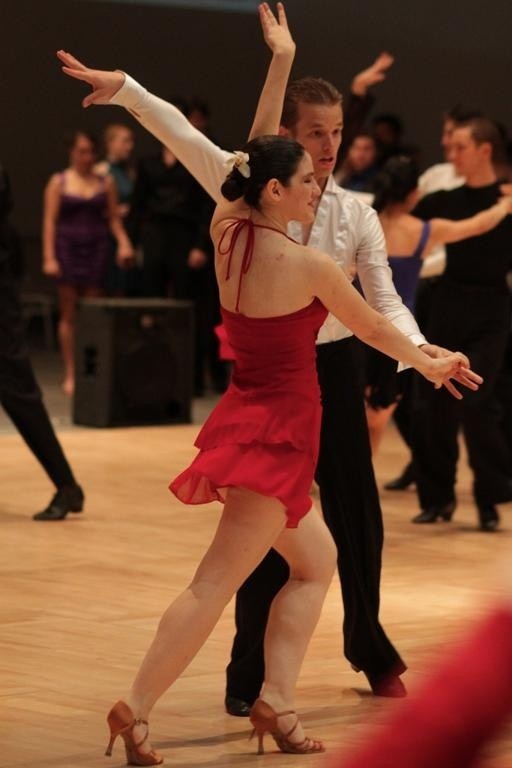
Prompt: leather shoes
<box><xmin>34</xmin><ymin>484</ymin><xmax>84</xmax><ymax>520</ymax></box>
<box><xmin>382</xmin><ymin>480</ymin><xmax>499</xmax><ymax>530</ymax></box>
<box><xmin>350</xmin><ymin>661</ymin><xmax>407</xmax><ymax>697</ymax></box>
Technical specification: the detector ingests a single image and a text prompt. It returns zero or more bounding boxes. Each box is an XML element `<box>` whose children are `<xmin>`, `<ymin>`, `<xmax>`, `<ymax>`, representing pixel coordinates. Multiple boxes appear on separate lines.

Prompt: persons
<box><xmin>39</xmin><ymin>105</ymin><xmax>221</xmax><ymax>398</ymax></box>
<box><xmin>362</xmin><ymin>159</ymin><xmax>512</xmax><ymax>455</ymax></box>
<box><xmin>1</xmin><ymin>164</ymin><xmax>84</xmax><ymax>523</ymax></box>
<box><xmin>333</xmin><ymin>56</ymin><xmax>417</xmax><ymax>216</ymax></box>
<box><xmin>54</xmin><ymin>46</ymin><xmax>484</xmax><ymax>720</ymax></box>
<box><xmin>407</xmin><ymin>122</ymin><xmax>511</xmax><ymax>534</ymax></box>
<box><xmin>384</xmin><ymin>111</ymin><xmax>483</xmax><ymax>490</ymax></box>
<box><xmin>106</xmin><ymin>0</ymin><xmax>468</xmax><ymax>768</ymax></box>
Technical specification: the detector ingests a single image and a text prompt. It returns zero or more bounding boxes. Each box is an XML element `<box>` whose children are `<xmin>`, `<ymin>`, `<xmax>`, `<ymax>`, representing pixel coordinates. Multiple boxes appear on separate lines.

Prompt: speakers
<box><xmin>72</xmin><ymin>297</ymin><xmax>193</xmax><ymax>427</ymax></box>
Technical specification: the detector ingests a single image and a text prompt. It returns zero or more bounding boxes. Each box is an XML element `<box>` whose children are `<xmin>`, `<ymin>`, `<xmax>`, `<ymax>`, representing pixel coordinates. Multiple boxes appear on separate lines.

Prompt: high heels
<box><xmin>250</xmin><ymin>697</ymin><xmax>325</xmax><ymax>753</ymax></box>
<box><xmin>105</xmin><ymin>703</ymin><xmax>163</xmax><ymax>766</ymax></box>
